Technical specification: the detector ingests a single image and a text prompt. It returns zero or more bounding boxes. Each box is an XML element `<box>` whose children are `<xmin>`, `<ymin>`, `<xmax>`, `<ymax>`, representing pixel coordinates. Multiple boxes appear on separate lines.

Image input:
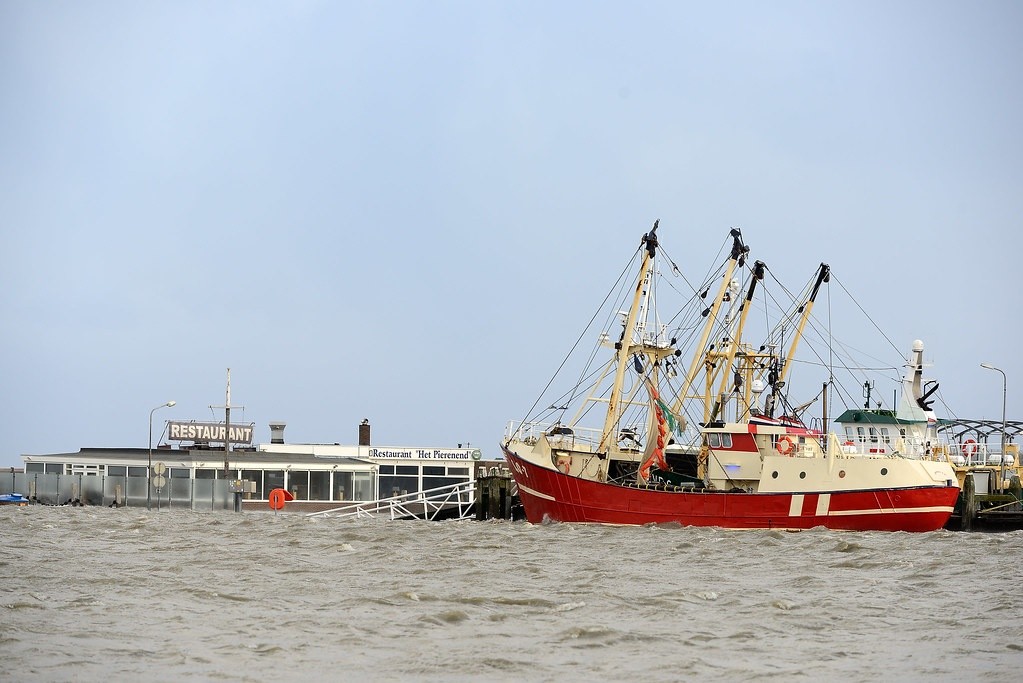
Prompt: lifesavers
<box><xmin>962</xmin><ymin>440</ymin><xmax>976</xmax><ymax>457</ymax></box>
<box><xmin>777</xmin><ymin>437</ymin><xmax>793</xmax><ymax>454</ymax></box>
<box><xmin>556</xmin><ymin>460</ymin><xmax>569</xmax><ymax>475</ymax></box>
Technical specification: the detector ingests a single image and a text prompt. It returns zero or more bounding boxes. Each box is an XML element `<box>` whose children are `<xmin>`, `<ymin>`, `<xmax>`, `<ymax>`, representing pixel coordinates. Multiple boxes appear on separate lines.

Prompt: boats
<box><xmin>501</xmin><ymin>218</ymin><xmax>961</xmax><ymax>534</ymax></box>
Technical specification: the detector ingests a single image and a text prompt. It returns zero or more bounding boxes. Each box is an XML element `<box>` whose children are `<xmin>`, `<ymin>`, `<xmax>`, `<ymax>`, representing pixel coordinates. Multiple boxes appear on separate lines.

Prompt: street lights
<box><xmin>147</xmin><ymin>402</ymin><xmax>175</xmax><ymax>511</ymax></box>
<box><xmin>980</xmin><ymin>362</ymin><xmax>1007</xmax><ymax>494</ymax></box>
